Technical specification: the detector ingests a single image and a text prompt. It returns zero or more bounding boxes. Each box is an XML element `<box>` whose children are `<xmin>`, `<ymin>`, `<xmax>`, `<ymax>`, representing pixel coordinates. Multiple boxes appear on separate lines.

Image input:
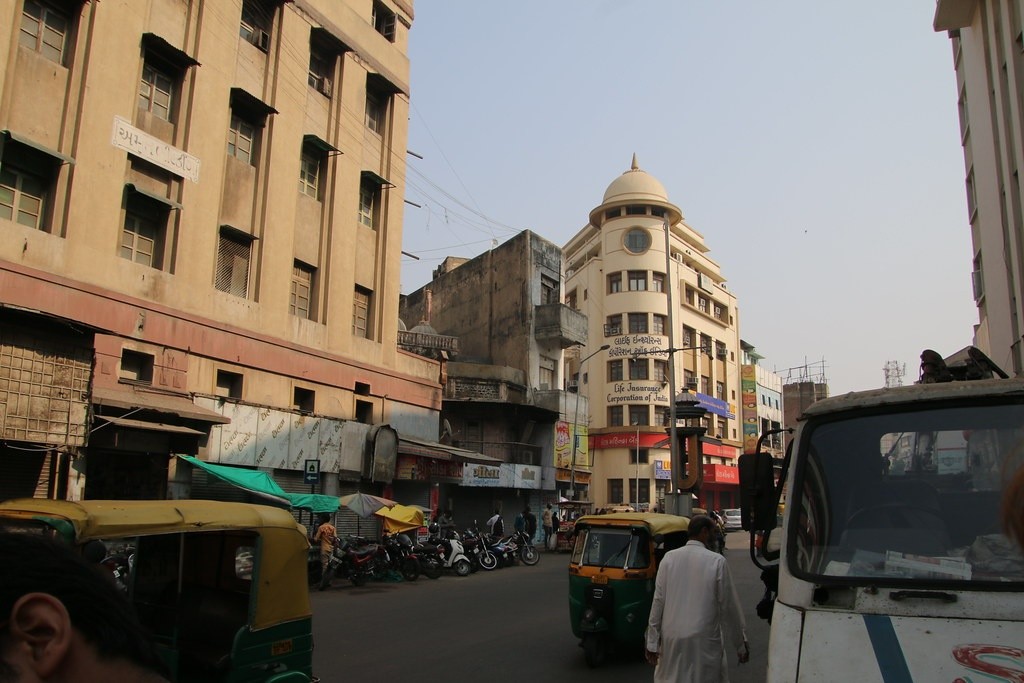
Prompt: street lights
<box><xmin>569</xmin><ymin>345</ymin><xmax>611</xmax><ymax>501</ymax></box>
<box><xmin>633</xmin><ymin>422</ymin><xmax>639</xmax><ymax>512</ymax></box>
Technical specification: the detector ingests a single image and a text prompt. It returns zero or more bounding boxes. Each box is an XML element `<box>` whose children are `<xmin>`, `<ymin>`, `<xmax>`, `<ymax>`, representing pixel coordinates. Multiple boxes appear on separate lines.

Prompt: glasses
<box><xmin>548</xmin><ymin>506</ymin><xmax>553</xmax><ymax>507</ymax></box>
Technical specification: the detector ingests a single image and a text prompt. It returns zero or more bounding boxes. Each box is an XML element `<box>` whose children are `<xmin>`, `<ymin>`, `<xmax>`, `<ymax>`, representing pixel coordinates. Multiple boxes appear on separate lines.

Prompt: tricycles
<box><xmin>555</xmin><ymin>501</ymin><xmax>594</xmax><ymax>554</ymax></box>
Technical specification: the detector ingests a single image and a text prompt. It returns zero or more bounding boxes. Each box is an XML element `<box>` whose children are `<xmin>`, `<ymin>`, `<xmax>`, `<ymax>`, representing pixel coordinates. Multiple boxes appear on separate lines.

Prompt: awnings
<box><xmin>398</xmin><ymin>433</ymin><xmax>503</xmax><ymax>466</ymax></box>
<box><xmin>90</xmin><ymin>387</ymin><xmax>231</xmax><ymax>435</ymax></box>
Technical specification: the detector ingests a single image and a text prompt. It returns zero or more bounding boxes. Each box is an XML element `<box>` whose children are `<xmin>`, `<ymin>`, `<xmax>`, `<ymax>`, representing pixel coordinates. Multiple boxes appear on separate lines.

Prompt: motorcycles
<box><xmin>568</xmin><ymin>509</ymin><xmax>691</xmax><ymax>656</ymax></box>
<box><xmin>1</xmin><ymin>496</ymin><xmax>321</xmax><ymax>683</ymax></box>
<box><xmin>320</xmin><ymin>519</ymin><xmax>540</xmax><ymax>585</ymax></box>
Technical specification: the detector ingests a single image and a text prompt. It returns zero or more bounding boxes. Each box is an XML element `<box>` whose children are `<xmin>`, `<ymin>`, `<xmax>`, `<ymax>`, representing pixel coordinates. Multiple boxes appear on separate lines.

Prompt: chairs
<box><xmin>841</xmin><ymin>469</ymin><xmax>960</xmax><ymax>558</ymax></box>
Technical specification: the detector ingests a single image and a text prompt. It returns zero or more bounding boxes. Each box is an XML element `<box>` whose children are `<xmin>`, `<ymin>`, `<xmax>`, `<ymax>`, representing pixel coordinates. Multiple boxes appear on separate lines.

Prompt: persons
<box><xmin>0</xmin><ymin>534</ymin><xmax>171</xmax><ymax>683</ymax></box>
<box><xmin>542</xmin><ymin>502</ymin><xmax>559</xmax><ymax>551</ymax></box>
<box><xmin>313</xmin><ymin>512</ymin><xmax>338</xmax><ymax>591</ymax></box>
<box><xmin>592</xmin><ymin>508</ymin><xmax>606</xmax><ymax>515</ymax></box>
<box><xmin>916</xmin><ymin>350</ymin><xmax>954</xmax><ymax>383</ymax></box>
<box><xmin>514</xmin><ymin>506</ymin><xmax>536</xmax><ymax>545</ymax></box>
<box><xmin>487</xmin><ymin>508</ymin><xmax>504</xmax><ymax>535</ymax></box>
<box><xmin>710</xmin><ymin>511</ymin><xmax>728</xmax><ymax>553</ymax></box>
<box><xmin>645</xmin><ymin>515</ymin><xmax>750</xmax><ymax>683</ymax></box>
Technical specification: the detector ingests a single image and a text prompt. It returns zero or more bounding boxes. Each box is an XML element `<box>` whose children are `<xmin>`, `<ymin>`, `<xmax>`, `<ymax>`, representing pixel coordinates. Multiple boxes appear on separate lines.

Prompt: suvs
<box><xmin>720</xmin><ymin>508</ymin><xmax>742</xmax><ymax>531</ymax></box>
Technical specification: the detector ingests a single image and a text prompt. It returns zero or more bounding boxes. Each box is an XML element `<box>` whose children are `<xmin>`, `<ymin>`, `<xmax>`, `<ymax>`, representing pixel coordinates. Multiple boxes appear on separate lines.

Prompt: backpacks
<box><xmin>493</xmin><ymin>516</ymin><xmax>503</xmax><ymax>537</ymax></box>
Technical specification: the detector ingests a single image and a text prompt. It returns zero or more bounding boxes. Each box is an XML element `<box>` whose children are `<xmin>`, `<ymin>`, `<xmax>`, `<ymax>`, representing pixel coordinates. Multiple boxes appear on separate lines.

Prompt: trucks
<box><xmin>738</xmin><ymin>376</ymin><xmax>1023</xmax><ymax>683</ymax></box>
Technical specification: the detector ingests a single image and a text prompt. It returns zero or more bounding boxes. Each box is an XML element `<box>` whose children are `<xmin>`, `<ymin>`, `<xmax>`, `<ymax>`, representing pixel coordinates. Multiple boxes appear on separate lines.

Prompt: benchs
<box><xmin>147</xmin><ymin>579</ymin><xmax>250</xmax><ymax>683</ymax></box>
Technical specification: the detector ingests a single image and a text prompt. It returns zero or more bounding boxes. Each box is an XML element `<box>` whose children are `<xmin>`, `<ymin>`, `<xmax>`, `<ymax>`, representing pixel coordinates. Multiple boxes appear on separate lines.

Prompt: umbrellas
<box><xmin>339</xmin><ymin>490</ymin><xmax>398</xmax><ymax>536</ymax></box>
